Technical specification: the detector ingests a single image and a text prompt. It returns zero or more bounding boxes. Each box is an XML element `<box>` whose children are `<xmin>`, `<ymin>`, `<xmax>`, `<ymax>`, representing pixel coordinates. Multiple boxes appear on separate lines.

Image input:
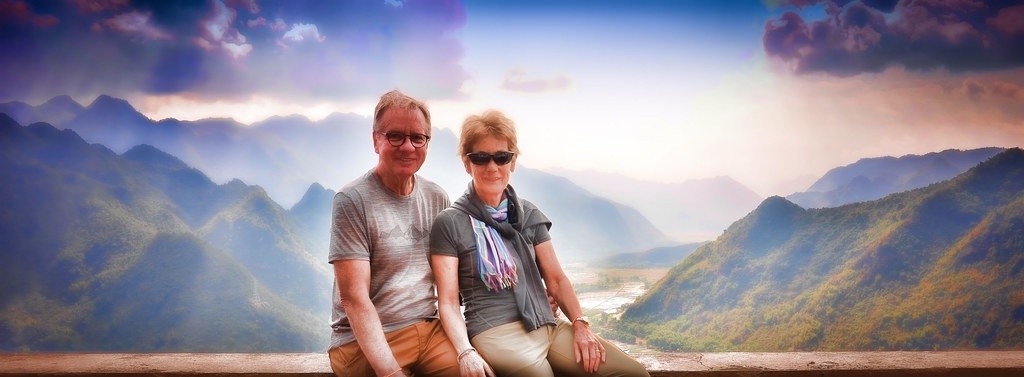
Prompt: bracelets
<box><xmin>456</xmin><ymin>347</ymin><xmax>479</xmax><ymax>365</ymax></box>
<box><xmin>383</xmin><ymin>367</ymin><xmax>403</xmax><ymax>377</ymax></box>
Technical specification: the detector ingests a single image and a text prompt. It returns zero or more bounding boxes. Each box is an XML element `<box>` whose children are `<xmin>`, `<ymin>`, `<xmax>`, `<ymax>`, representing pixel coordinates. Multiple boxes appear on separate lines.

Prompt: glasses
<box><xmin>377</xmin><ymin>129</ymin><xmax>430</xmax><ymax>147</ymax></box>
<box><xmin>466</xmin><ymin>151</ymin><xmax>516</xmax><ymax>165</ymax></box>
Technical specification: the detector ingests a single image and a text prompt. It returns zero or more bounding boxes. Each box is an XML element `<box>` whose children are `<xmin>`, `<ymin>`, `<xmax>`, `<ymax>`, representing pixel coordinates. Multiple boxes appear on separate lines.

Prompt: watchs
<box><xmin>572</xmin><ymin>316</ymin><xmax>590</xmax><ymax>328</ymax></box>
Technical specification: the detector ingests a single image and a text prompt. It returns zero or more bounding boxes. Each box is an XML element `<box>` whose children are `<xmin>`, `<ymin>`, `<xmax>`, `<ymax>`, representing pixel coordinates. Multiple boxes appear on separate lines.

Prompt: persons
<box><xmin>327</xmin><ymin>92</ymin><xmax>460</xmax><ymax>377</ymax></box>
<box><xmin>431</xmin><ymin>110</ymin><xmax>651</xmax><ymax>377</ymax></box>
